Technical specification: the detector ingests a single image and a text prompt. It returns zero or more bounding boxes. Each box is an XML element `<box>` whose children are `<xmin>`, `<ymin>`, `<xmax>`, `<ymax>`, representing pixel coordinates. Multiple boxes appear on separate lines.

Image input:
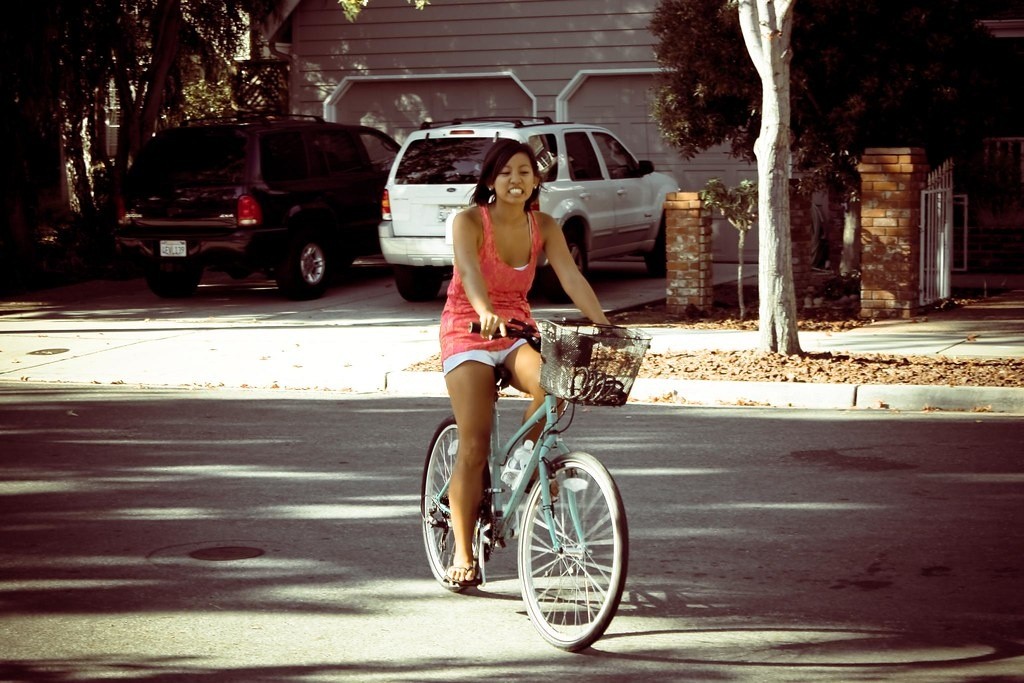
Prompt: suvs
<box><xmin>381</xmin><ymin>114</ymin><xmax>684</xmax><ymax>303</ymax></box>
<box><xmin>117</xmin><ymin>115</ymin><xmax>404</xmax><ymax>302</ymax></box>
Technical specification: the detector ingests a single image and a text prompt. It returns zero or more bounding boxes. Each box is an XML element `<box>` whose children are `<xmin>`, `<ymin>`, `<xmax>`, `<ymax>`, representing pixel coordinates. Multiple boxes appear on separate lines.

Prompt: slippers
<box><xmin>446</xmin><ymin>565</ymin><xmax>478</xmax><ymax>586</ymax></box>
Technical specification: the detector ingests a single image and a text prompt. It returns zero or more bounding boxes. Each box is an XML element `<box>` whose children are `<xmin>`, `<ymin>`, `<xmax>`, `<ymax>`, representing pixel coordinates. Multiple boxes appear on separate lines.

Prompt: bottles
<box><xmin>502</xmin><ymin>440</ymin><xmax>534</xmax><ymax>486</ymax></box>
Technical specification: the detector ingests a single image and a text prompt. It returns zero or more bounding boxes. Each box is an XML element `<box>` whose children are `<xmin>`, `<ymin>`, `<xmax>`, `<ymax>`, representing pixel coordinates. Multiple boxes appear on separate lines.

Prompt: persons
<box><xmin>437</xmin><ymin>139</ymin><xmax>632</xmax><ymax>585</ymax></box>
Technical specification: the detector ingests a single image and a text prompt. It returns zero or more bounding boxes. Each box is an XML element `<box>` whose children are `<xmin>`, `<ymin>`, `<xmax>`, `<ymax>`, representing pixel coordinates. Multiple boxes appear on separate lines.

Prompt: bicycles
<box><xmin>419</xmin><ymin>306</ymin><xmax>654</xmax><ymax>652</ymax></box>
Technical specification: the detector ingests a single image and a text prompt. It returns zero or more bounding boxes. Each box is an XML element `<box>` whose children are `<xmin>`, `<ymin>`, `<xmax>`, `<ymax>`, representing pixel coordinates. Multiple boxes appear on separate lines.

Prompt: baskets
<box><xmin>537</xmin><ymin>318</ymin><xmax>652</xmax><ymax>408</ymax></box>
